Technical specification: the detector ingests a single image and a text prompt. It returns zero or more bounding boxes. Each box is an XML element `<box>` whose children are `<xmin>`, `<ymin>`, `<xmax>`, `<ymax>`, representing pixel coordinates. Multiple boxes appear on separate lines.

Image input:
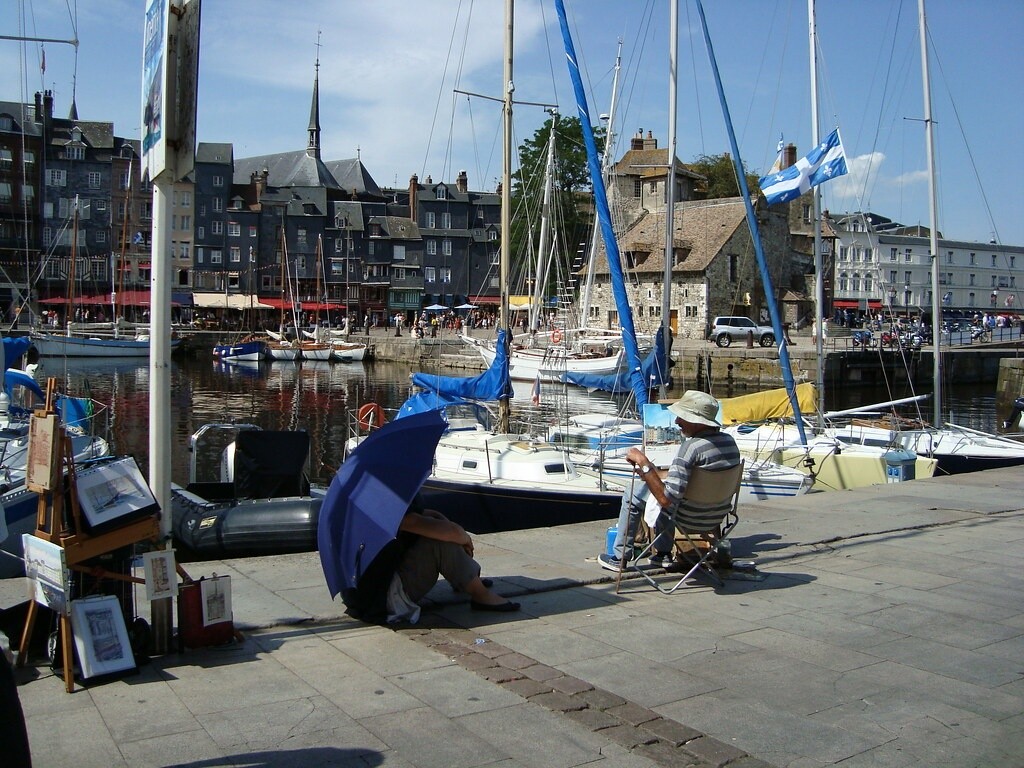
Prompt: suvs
<box><xmin>706</xmin><ymin>315</ymin><xmax>776</xmax><ymax>348</ymax></box>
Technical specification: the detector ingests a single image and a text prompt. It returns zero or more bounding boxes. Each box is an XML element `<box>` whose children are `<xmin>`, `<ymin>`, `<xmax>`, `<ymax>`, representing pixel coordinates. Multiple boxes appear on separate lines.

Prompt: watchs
<box><xmin>641</xmin><ymin>464</ymin><xmax>654</xmax><ymax>476</ymax></box>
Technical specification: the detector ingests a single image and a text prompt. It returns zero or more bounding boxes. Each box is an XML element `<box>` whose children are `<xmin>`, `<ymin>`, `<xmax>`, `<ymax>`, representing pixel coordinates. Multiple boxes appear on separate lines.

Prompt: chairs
<box><xmin>632</xmin><ymin>458</ymin><xmax>745</xmax><ymax>595</ymax></box>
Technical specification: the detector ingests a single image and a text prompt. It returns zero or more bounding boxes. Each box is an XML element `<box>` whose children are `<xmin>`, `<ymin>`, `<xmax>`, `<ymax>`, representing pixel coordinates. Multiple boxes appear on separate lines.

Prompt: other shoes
<box><xmin>598</xmin><ymin>554</ymin><xmax>627</xmax><ymax>571</ymax></box>
<box><xmin>451</xmin><ymin>578</ymin><xmax>493</xmax><ymax>594</ymax></box>
<box><xmin>471</xmin><ymin>599</ymin><xmax>520</xmax><ymax>610</ymax></box>
<box><xmin>648</xmin><ymin>554</ymin><xmax>674</xmax><ymax>567</ymax></box>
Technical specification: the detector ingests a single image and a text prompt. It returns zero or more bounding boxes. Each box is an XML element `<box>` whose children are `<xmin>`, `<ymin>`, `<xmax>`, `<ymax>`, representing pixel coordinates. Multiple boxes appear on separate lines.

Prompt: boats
<box><xmin>170</xmin><ymin>416</ymin><xmax>338</xmax><ymax>561</ymax></box>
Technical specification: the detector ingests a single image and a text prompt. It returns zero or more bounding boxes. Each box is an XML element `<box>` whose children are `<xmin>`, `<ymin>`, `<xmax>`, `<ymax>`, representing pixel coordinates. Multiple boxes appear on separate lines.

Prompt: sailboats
<box><xmin>339</xmin><ymin>0</ymin><xmax>1024</xmax><ymax>534</ymax></box>
<box><xmin>314</xmin><ymin>210</ymin><xmax>368</xmax><ymax>362</ymax></box>
<box><xmin>1</xmin><ymin>0</ymin><xmax>185</xmax><ymax>651</ymax></box>
<box><xmin>293</xmin><ymin>233</ymin><xmax>336</xmax><ymax>362</ymax></box>
<box><xmin>212</xmin><ymin>245</ymin><xmax>269</xmax><ymax>362</ymax></box>
<box><xmin>238</xmin><ymin>205</ymin><xmax>305</xmax><ymax>362</ymax></box>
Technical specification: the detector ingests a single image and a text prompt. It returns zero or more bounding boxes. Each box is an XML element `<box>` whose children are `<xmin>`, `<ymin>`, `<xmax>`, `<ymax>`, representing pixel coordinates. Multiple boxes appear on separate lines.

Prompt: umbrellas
<box><xmin>421</xmin><ymin>303</ymin><xmax>451</xmax><ymax>310</ymax></box>
<box><xmin>315</xmin><ymin>407</ymin><xmax>450</xmax><ymax>602</ymax></box>
<box><xmin>454</xmin><ymin>302</ymin><xmax>480</xmax><ymax>310</ymax></box>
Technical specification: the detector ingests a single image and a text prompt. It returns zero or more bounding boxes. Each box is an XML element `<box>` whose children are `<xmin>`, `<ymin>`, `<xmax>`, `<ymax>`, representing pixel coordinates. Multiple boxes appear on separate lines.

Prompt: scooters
<box><xmin>969</xmin><ymin>321</ymin><xmax>989</xmax><ymax>343</ymax></box>
<box><xmin>880</xmin><ymin>324</ymin><xmax>901</xmax><ymax>347</ymax></box>
<box><xmin>850</xmin><ymin>325</ymin><xmax>878</xmax><ymax>348</ymax></box>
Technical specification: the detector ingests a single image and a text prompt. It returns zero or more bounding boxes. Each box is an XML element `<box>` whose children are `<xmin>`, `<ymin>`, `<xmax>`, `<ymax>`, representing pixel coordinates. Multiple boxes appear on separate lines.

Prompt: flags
<box><xmin>758</xmin><ymin>129</ymin><xmax>849</xmax><ymax>208</ymax></box>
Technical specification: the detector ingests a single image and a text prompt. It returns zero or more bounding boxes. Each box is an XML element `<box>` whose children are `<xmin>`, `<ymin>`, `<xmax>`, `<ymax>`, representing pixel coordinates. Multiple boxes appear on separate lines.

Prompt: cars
<box><xmin>878</xmin><ymin>317</ymin><xmax>910</xmax><ymax>333</ymax></box>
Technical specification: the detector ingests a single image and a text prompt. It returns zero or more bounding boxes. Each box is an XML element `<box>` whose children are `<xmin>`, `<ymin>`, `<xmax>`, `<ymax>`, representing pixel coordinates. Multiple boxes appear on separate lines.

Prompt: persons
<box><xmin>394</xmin><ymin>311</ymin><xmax>406</xmax><ymax>330</ymax></box>
<box><xmin>287</xmin><ymin>317</ymin><xmax>326</xmax><ymax>327</ymax></box>
<box><xmin>222</xmin><ymin>314</ymin><xmax>227</xmax><ymax>328</ymax></box>
<box><xmin>342</xmin><ymin>314</ymin><xmax>356</xmax><ymax>334</ymax></box>
<box><xmin>364</xmin><ymin>313</ymin><xmax>377</xmax><ymax>329</ymax></box>
<box><xmin>971</xmin><ymin>313</ymin><xmax>995</xmax><ymax>337</ymax></box>
<box><xmin>340</xmin><ymin>489</ymin><xmax>521</xmax><ymax>616</ymax></box>
<box><xmin>522</xmin><ymin>313</ymin><xmax>543</xmax><ymax>333</ymax></box>
<box><xmin>47</xmin><ymin>310</ymin><xmax>58</xmax><ymax>329</ymax></box>
<box><xmin>833</xmin><ymin>307</ymin><xmax>882</xmax><ymax>328</ymax></box>
<box><xmin>598</xmin><ymin>390</ymin><xmax>740</xmax><ymax>572</ymax></box>
<box><xmin>76</xmin><ymin>308</ymin><xmax>124</xmax><ymax>327</ymax></box>
<box><xmin>1000</xmin><ymin>316</ymin><xmax>1020</xmax><ymax>328</ymax></box>
<box><xmin>413</xmin><ymin>310</ymin><xmax>496</xmax><ymax>340</ymax></box>
<box><xmin>15</xmin><ymin>306</ymin><xmax>21</xmax><ymax>324</ymax></box>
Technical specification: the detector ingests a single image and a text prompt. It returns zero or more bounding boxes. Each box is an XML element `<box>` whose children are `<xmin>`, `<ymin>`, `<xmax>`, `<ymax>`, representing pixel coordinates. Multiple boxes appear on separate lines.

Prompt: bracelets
<box><xmin>465</xmin><ymin>534</ymin><xmax>468</xmax><ymax>544</ymax></box>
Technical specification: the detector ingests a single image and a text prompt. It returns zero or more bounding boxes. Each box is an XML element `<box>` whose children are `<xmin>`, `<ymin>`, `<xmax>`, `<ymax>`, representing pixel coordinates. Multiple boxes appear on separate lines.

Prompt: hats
<box><xmin>973</xmin><ymin>315</ymin><xmax>978</xmax><ymax>319</ymax></box>
<box><xmin>667</xmin><ymin>390</ymin><xmax>721</xmax><ymax>426</ymax></box>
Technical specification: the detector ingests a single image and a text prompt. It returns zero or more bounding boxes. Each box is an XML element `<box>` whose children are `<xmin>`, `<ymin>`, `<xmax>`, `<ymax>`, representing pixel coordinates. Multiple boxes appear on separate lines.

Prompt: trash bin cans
<box><xmin>703</xmin><ymin>325</ymin><xmax>711</xmax><ymax>340</ymax></box>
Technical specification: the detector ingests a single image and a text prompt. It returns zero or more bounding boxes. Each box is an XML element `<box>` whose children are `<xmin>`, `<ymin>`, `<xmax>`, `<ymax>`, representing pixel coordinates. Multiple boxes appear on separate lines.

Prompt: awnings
<box><xmin>37</xmin><ymin>298</ymin><xmax>177</xmax><ymax>306</ymax></box>
<box><xmin>833</xmin><ymin>301</ymin><xmax>882</xmax><ymax>309</ymax></box>
<box><xmin>271</xmin><ymin>303</ymin><xmax>348</xmax><ymax>309</ymax></box>
<box><xmin>198</xmin><ymin>303</ymin><xmax>275</xmax><ymax>310</ymax></box>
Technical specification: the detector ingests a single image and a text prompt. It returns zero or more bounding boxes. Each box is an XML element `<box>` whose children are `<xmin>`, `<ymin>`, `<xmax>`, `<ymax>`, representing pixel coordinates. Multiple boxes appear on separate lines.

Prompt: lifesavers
<box><xmin>552</xmin><ymin>330</ymin><xmax>561</xmax><ymax>343</ymax></box>
<box><xmin>358</xmin><ymin>403</ymin><xmax>385</xmax><ymax>430</ymax></box>
